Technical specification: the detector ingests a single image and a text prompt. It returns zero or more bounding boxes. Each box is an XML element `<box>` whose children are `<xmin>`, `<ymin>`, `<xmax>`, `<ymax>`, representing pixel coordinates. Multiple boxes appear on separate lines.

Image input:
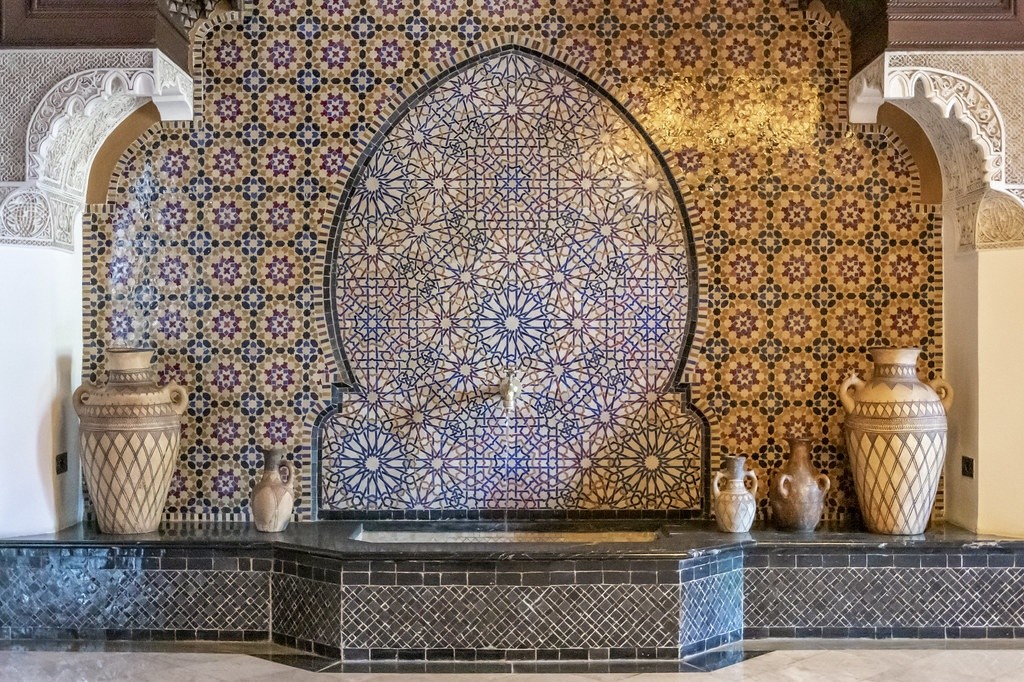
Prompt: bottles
<box><xmin>250</xmin><ymin>449</ymin><xmax>294</xmax><ymax>532</ymax></box>
<box><xmin>769</xmin><ymin>437</ymin><xmax>831</xmax><ymax>533</ymax></box>
<box><xmin>839</xmin><ymin>347</ymin><xmax>954</xmax><ymax>536</ymax></box>
<box><xmin>712</xmin><ymin>456</ymin><xmax>758</xmax><ymax>533</ymax></box>
<box><xmin>72</xmin><ymin>348</ymin><xmax>189</xmax><ymax>535</ymax></box>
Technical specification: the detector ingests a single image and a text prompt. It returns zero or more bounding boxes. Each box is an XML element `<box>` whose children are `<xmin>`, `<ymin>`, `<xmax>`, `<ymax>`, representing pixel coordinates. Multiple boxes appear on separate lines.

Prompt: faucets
<box><xmin>501</xmin><ymin>369</ymin><xmax>523</xmax><ymax>410</ymax></box>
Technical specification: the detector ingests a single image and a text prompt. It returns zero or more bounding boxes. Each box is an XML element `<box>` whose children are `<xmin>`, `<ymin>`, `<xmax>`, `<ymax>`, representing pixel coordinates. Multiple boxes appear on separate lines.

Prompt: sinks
<box><xmin>346</xmin><ymin>520</ymin><xmax>662</xmax><ymax>546</ymax></box>
